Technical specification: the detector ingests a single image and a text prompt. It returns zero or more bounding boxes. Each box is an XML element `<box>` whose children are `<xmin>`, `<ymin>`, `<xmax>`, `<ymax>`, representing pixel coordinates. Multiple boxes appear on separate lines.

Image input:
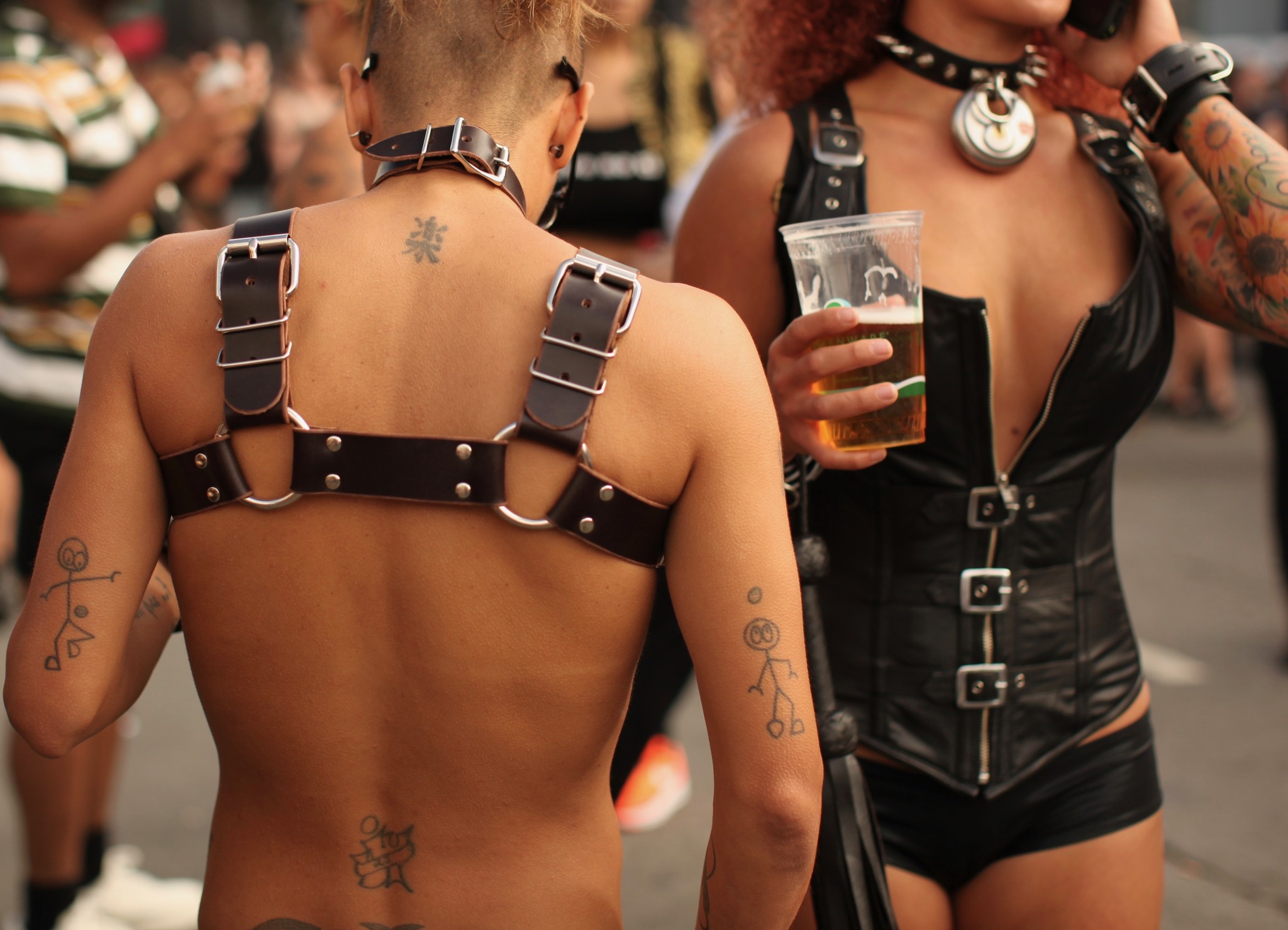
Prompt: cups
<box><xmin>780</xmin><ymin>209</ymin><xmax>927</xmax><ymax>453</ymax></box>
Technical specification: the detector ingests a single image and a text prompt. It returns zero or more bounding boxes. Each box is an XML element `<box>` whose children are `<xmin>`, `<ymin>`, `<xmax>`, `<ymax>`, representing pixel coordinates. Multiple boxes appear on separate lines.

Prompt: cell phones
<box><xmin>1066</xmin><ymin>0</ymin><xmax>1132</xmax><ymax>40</ymax></box>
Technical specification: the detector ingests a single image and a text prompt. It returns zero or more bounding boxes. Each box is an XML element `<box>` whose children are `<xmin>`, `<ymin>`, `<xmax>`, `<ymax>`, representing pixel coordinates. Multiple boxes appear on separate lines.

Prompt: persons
<box><xmin>3</xmin><ymin>0</ymin><xmax>738</xmax><ymax>329</ymax></box>
<box><xmin>4</xmin><ymin>0</ymin><xmax>288</xmax><ymax>930</ymax></box>
<box><xmin>2</xmin><ymin>1</ymin><xmax>819</xmax><ymax>930</ymax></box>
<box><xmin>658</xmin><ymin>1</ymin><xmax>1287</xmax><ymax>930</ymax></box>
<box><xmin>1132</xmin><ymin>67</ymin><xmax>1287</xmax><ymax>609</ymax></box>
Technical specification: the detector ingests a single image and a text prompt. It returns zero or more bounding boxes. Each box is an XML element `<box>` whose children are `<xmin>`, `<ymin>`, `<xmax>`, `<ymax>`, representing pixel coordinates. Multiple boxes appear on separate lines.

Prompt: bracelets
<box><xmin>1119</xmin><ymin>29</ymin><xmax>1243</xmax><ymax>156</ymax></box>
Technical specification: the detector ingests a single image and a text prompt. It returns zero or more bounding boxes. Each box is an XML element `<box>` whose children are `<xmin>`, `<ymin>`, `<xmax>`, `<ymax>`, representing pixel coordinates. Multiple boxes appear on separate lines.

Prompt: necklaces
<box><xmin>863</xmin><ymin>14</ymin><xmax>1051</xmax><ymax>173</ymax></box>
